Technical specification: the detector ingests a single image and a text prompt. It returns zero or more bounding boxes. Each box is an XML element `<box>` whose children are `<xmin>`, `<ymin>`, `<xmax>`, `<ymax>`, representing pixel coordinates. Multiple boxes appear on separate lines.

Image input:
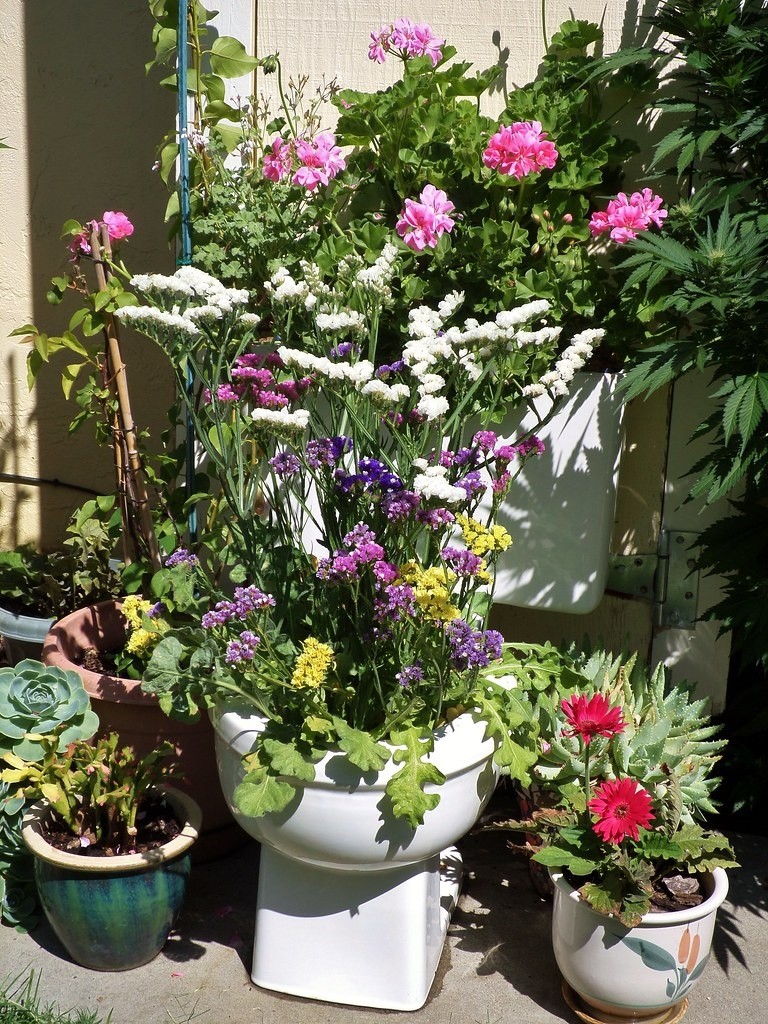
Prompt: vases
<box><xmin>22</xmin><ymin>781</ymin><xmax>202</xmax><ymax>973</ymax></box>
<box><xmin>42</xmin><ymin>594</ymin><xmax>252</xmax><ymax>866</ymax></box>
<box><xmin>548</xmin><ymin>856</ymin><xmax>729</xmax><ymax>1024</ymax></box>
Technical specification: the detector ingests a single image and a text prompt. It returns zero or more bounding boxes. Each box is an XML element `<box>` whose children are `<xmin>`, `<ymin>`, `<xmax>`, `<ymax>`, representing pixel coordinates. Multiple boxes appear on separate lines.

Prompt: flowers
<box><xmin>16</xmin><ymin>208</ymin><xmax>172</xmax><ymax>603</ymax></box>
<box><xmin>65</xmin><ymin>17</ymin><xmax>675</xmax><ymax>425</ymax></box>
<box><xmin>24</xmin><ymin>724</ymin><xmax>182</xmax><ymax>852</ymax></box>
<box><xmin>115</xmin><ymin>239</ymin><xmax>598</xmax><ymax>831</ymax></box>
<box><xmin>531</xmin><ymin>691</ymin><xmax>741</xmax><ymax>928</ymax></box>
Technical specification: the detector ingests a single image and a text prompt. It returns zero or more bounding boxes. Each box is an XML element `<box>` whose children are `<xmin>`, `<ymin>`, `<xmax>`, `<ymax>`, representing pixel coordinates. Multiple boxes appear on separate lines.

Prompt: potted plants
<box><xmin>511</xmin><ymin>647</ymin><xmax>729</xmax><ymax>902</ymax></box>
<box><xmin>0</xmin><ymin>499</ymin><xmax>130</xmax><ymax>667</ymax></box>
